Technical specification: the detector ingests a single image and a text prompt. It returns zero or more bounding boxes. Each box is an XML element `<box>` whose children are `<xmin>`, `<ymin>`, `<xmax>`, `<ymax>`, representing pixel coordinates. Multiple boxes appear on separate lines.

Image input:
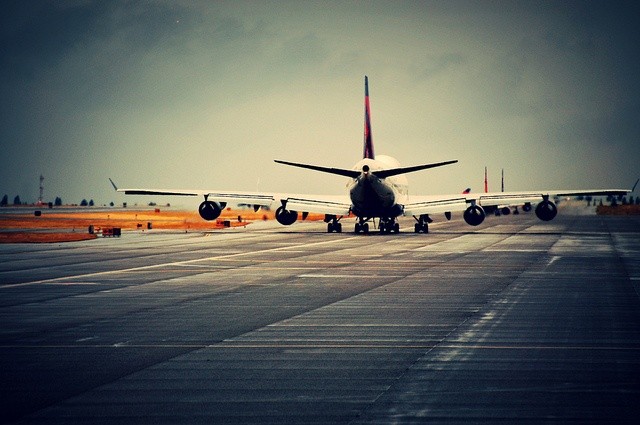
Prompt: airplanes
<box><xmin>108</xmin><ymin>76</ymin><xmax>633</xmax><ymax>235</ymax></box>
